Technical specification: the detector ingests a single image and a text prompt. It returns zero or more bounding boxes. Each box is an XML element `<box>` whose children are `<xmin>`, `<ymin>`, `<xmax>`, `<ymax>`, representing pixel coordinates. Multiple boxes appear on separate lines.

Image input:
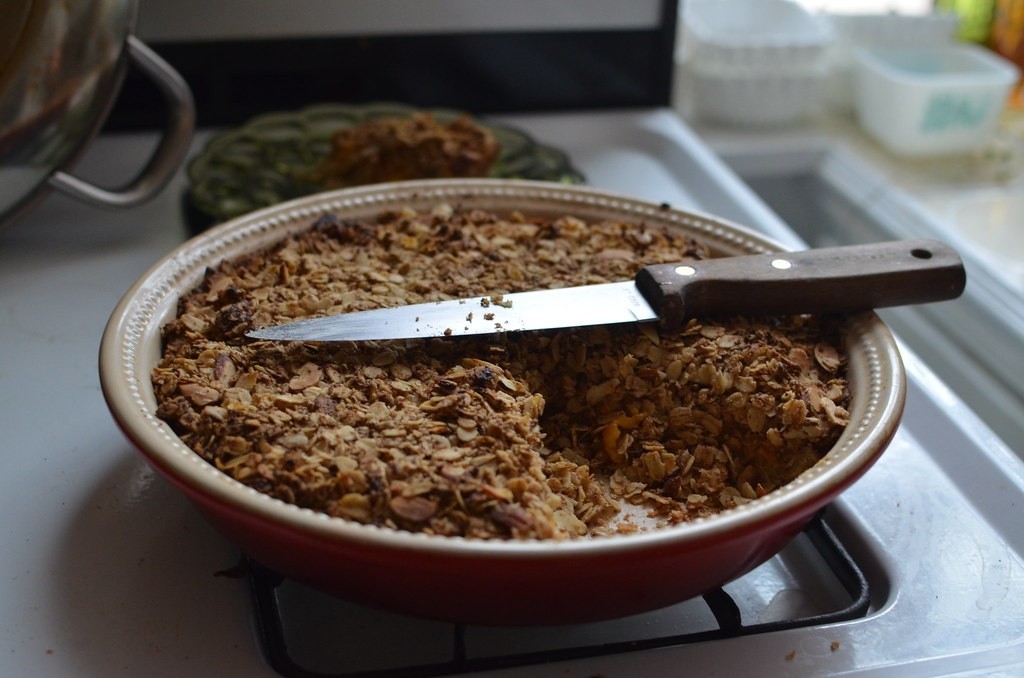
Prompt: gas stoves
<box><xmin>1</xmin><ymin>0</ymin><xmax>1023</xmax><ymax>678</ymax></box>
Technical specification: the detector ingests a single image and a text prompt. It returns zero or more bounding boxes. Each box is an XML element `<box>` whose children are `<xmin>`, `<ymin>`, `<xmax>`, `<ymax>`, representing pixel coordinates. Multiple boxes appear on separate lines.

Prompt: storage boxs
<box><xmin>679</xmin><ymin>63</ymin><xmax>833</xmax><ymax>132</ymax></box>
<box><xmin>677</xmin><ymin>0</ymin><xmax>833</xmax><ymax>66</ymax></box>
<box><xmin>847</xmin><ymin>39</ymin><xmax>1021</xmax><ymax>157</ymax></box>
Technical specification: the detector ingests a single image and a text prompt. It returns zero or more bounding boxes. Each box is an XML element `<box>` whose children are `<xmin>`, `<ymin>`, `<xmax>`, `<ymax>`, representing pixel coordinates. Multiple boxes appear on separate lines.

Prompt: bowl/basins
<box><xmin>97</xmin><ymin>174</ymin><xmax>907</xmax><ymax>616</ymax></box>
<box><xmin>672</xmin><ymin>1</ymin><xmax>1020</xmax><ymax>155</ymax></box>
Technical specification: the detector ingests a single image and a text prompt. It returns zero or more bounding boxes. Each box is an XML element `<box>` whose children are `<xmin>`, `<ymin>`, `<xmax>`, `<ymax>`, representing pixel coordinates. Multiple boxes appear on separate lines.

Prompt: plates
<box><xmin>187</xmin><ymin>105</ymin><xmax>587</xmax><ymax>220</ymax></box>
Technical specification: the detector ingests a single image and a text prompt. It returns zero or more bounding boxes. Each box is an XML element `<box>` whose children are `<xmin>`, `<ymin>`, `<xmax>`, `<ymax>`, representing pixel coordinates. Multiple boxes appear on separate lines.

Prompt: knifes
<box><xmin>246</xmin><ymin>239</ymin><xmax>966</xmax><ymax>341</ymax></box>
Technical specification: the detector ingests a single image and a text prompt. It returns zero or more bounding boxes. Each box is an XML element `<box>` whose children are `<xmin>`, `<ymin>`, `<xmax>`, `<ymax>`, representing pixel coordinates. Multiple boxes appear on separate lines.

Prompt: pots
<box><xmin>0</xmin><ymin>0</ymin><xmax>196</xmax><ymax>233</ymax></box>
